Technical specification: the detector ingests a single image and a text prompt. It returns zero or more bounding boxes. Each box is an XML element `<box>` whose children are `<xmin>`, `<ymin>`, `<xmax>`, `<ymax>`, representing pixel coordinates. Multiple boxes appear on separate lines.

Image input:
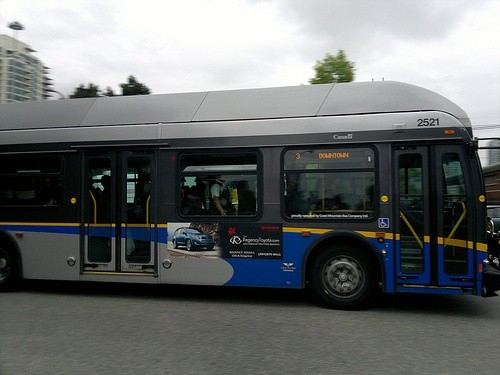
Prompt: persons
<box><xmin>85</xmin><ymin>154</ymin><xmax>458</xmax><ymax>266</ymax></box>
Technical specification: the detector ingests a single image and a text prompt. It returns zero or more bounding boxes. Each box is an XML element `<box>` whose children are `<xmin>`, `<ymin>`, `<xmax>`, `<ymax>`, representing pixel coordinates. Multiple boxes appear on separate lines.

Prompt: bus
<box><xmin>0</xmin><ymin>78</ymin><xmax>500</xmax><ymax>309</ymax></box>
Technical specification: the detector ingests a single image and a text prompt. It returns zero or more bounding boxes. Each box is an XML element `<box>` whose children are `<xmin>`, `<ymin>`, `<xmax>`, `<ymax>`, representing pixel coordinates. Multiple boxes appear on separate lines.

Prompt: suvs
<box><xmin>172</xmin><ymin>227</ymin><xmax>215</xmax><ymax>251</ymax></box>
